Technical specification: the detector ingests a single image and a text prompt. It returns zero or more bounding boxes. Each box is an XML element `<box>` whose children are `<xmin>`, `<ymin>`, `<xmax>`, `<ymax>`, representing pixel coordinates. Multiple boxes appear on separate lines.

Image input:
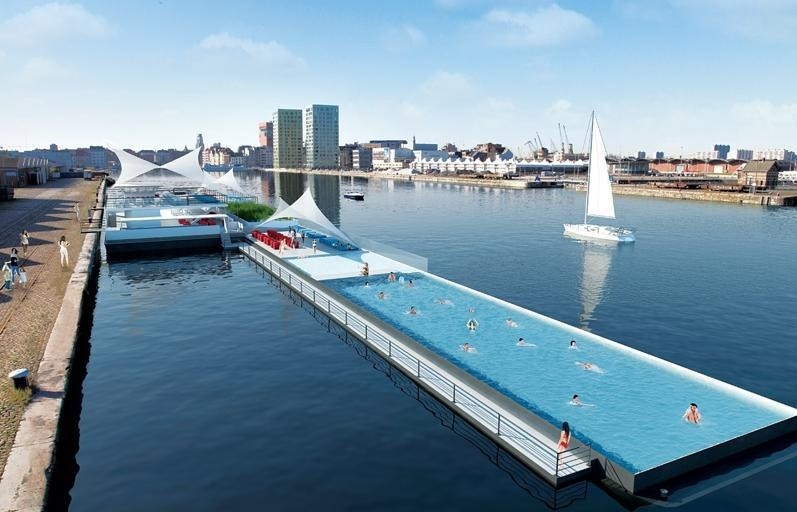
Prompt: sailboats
<box><xmin>562</xmin><ymin>110</ymin><xmax>636</xmax><ymax>243</ymax></box>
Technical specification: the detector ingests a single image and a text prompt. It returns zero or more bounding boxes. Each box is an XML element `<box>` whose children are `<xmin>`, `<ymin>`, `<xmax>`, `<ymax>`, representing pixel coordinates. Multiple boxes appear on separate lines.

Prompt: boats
<box><xmin>343</xmin><ymin>190</ymin><xmax>364</xmax><ymax>200</ymax></box>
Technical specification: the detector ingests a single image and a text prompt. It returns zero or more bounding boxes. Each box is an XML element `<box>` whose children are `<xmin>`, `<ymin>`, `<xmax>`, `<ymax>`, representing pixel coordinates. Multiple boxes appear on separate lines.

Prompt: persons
<box><xmin>555</xmin><ymin>420</ymin><xmax>571</xmax><ymax>466</ymax></box>
<box><xmin>681</xmin><ymin>403</ymin><xmax>700</xmax><ymax>426</ymax></box>
<box><xmin>58</xmin><ymin>235</ymin><xmax>70</xmax><ymax>268</ymax></box>
<box><xmin>4</xmin><ymin>247</ymin><xmax>22</xmax><ymax>285</ymax></box>
<box><xmin>278</xmin><ymin>225</ymin><xmax>352</xmax><ymax>258</ymax></box>
<box><xmin>358</xmin><ymin>262</ymin><xmax>604</xmax><ymax>413</ymax></box>
<box><xmin>73</xmin><ymin>201</ymin><xmax>81</xmax><ymax>222</ymax></box>
<box><xmin>19</xmin><ymin>229</ymin><xmax>29</xmax><ymax>257</ymax></box>
<box><xmin>17</xmin><ymin>267</ymin><xmax>27</xmax><ymax>289</ymax></box>
<box><xmin>3</xmin><ymin>268</ymin><xmax>11</xmax><ymax>292</ymax></box>
